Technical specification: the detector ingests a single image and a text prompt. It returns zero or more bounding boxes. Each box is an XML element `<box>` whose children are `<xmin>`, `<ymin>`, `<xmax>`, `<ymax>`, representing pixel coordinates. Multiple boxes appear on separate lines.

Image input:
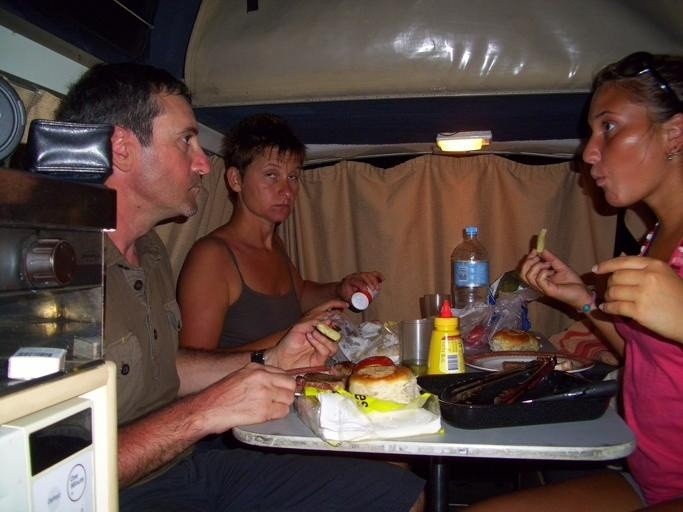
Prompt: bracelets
<box><xmin>250</xmin><ymin>351</ymin><xmax>265</xmax><ymax>365</ymax></box>
<box><xmin>575</xmin><ymin>290</ymin><xmax>598</xmax><ymax>314</ymax></box>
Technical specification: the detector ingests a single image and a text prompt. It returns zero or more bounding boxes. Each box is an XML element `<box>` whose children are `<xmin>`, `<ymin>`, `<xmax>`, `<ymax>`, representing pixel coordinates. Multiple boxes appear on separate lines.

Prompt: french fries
<box><xmin>317</xmin><ymin>323</ymin><xmax>341</xmax><ymax>342</ymax></box>
<box><xmin>534</xmin><ymin>228</ymin><xmax>547</xmax><ymax>251</ymax></box>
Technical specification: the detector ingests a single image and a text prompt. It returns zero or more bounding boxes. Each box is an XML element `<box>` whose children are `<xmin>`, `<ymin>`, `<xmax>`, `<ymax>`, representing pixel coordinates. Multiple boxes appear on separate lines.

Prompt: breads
<box><xmin>490</xmin><ymin>327</ymin><xmax>539</xmax><ymax>352</ymax></box>
<box><xmin>346</xmin><ymin>355</ymin><xmax>420</xmax><ymax>405</ymax></box>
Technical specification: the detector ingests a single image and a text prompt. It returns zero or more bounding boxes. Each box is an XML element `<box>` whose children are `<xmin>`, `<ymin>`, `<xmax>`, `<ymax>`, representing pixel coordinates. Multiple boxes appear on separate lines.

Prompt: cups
<box><xmin>398</xmin><ymin>318</ymin><xmax>433</xmax><ymax>368</ymax></box>
<box><xmin>422</xmin><ymin>292</ymin><xmax>448</xmax><ymax>316</ymax></box>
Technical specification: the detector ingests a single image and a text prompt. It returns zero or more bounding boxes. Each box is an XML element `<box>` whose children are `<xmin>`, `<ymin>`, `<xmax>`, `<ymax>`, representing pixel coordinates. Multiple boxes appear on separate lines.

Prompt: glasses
<box><xmin>617</xmin><ymin>51</ymin><xmax>683</xmax><ymax>114</ymax></box>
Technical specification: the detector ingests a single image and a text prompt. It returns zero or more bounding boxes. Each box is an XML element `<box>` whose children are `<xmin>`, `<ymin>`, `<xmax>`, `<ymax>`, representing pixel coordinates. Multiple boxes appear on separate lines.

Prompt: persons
<box><xmin>54</xmin><ymin>61</ymin><xmax>431</xmax><ymax>510</ymax></box>
<box><xmin>465</xmin><ymin>51</ymin><xmax>683</xmax><ymax>512</ymax></box>
<box><xmin>175</xmin><ymin>114</ymin><xmax>385</xmax><ymax>356</ymax></box>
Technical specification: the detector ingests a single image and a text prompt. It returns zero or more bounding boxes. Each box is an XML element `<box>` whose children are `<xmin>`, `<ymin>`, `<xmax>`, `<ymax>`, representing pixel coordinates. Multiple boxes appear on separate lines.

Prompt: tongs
<box><xmin>440</xmin><ymin>355</ymin><xmax>560</xmax><ymax>405</ymax></box>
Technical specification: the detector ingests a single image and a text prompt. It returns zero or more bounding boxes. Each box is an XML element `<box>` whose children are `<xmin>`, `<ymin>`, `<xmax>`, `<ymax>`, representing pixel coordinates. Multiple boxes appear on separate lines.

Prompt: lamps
<box><xmin>434</xmin><ymin>126</ymin><xmax>493</xmax><ymax>153</ymax></box>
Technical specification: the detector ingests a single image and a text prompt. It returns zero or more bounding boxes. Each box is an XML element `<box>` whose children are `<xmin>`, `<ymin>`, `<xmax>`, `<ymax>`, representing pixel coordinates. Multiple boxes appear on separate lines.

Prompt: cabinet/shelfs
<box><xmin>0</xmin><ymin>172</ymin><xmax>118</xmax><ymax>397</ymax></box>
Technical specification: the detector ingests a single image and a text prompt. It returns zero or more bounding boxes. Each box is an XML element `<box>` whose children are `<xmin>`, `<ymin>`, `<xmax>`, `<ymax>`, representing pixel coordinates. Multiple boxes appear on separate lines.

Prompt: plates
<box><xmin>464</xmin><ymin>350</ymin><xmax>597</xmax><ymax>374</ymax></box>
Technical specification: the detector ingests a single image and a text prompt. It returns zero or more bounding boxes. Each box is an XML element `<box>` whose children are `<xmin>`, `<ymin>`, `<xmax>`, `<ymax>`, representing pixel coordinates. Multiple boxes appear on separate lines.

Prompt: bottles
<box><xmin>350</xmin><ymin>284</ymin><xmax>381</xmax><ymax>311</ymax></box>
<box><xmin>426</xmin><ymin>297</ymin><xmax>464</xmax><ymax>376</ymax></box>
<box><xmin>450</xmin><ymin>224</ymin><xmax>492</xmax><ymax>310</ymax></box>
